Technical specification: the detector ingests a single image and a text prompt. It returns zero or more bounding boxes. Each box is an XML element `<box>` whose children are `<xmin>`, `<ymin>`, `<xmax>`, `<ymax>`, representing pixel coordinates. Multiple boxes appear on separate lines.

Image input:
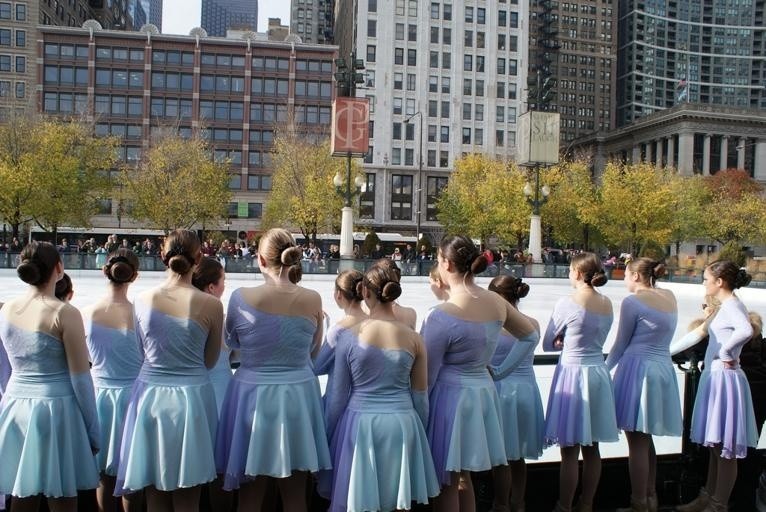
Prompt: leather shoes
<box><xmin>676</xmin><ymin>487</ymin><xmax>711</xmax><ymax>511</ymax></box>
<box><xmin>703</xmin><ymin>496</ymin><xmax>727</xmax><ymax>512</ymax></box>
<box><xmin>615</xmin><ymin>503</ymin><xmax>648</xmax><ymax>512</ymax></box>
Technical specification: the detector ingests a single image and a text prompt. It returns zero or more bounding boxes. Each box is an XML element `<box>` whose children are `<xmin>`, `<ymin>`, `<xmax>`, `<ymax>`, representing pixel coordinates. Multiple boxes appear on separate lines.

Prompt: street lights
<box><xmin>333</xmin><ymin>170</ymin><xmax>365</xmax><ymax>259</ymax></box>
<box><xmin>523</xmin><ymin>181</ymin><xmax>550</xmax><ymax>262</ymax></box>
<box><xmin>402</xmin><ymin>111</ymin><xmax>421</xmax><ymax>261</ymax></box>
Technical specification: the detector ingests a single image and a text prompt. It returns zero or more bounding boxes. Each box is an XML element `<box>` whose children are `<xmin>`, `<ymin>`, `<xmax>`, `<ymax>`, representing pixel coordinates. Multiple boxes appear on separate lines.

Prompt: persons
<box><xmin>0</xmin><ymin>223</ymin><xmax>765</xmax><ymax>512</ymax></box>
